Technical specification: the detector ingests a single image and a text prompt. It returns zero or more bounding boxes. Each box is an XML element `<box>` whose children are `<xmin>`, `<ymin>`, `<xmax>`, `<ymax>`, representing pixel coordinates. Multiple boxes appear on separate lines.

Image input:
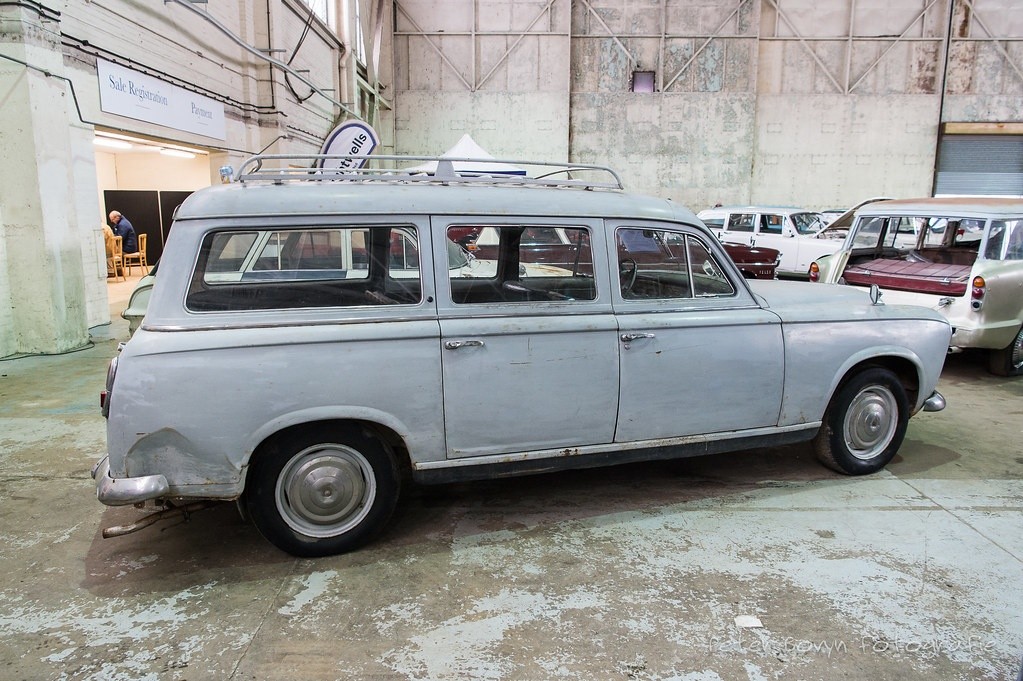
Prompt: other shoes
<box><xmin>117</xmin><ymin>270</ymin><xmax>123</xmax><ymax>276</ymax></box>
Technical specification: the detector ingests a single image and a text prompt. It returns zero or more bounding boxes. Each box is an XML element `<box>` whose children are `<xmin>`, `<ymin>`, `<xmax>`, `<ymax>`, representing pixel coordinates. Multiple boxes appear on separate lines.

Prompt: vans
<box><xmin>91</xmin><ymin>154</ymin><xmax>955</xmax><ymax>558</ymax></box>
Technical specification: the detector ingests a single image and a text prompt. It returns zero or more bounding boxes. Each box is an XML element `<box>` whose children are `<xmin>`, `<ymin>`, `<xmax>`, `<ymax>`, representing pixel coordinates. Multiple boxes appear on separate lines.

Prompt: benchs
<box><xmin>365</xmin><ymin>289</ymin><xmax>401</xmax><ymax>304</ymax></box>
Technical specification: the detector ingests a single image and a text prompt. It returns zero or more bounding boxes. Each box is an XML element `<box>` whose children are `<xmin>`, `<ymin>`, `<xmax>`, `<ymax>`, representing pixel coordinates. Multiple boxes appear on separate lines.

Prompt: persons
<box><xmin>108</xmin><ymin>210</ymin><xmax>137</xmax><ymax>278</ymax></box>
<box><xmin>101</xmin><ymin>222</ymin><xmax>116</xmax><ymax>258</ymax></box>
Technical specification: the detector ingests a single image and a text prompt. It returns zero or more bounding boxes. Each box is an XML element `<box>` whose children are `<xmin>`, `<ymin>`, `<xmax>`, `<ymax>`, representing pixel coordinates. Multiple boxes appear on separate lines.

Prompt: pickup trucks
<box><xmin>691</xmin><ymin>197</ymin><xmax>1023</xmax><ymax>378</ymax></box>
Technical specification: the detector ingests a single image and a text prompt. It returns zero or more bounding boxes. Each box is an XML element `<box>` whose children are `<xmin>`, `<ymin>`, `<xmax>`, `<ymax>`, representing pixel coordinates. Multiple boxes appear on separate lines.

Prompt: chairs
<box><xmin>106</xmin><ymin>235</ymin><xmax>127</xmax><ymax>282</ymax></box>
<box><xmin>123</xmin><ymin>234</ymin><xmax>150</xmax><ymax>278</ymax></box>
<box><xmin>502</xmin><ymin>280</ymin><xmax>575</xmax><ymax>301</ymax></box>
